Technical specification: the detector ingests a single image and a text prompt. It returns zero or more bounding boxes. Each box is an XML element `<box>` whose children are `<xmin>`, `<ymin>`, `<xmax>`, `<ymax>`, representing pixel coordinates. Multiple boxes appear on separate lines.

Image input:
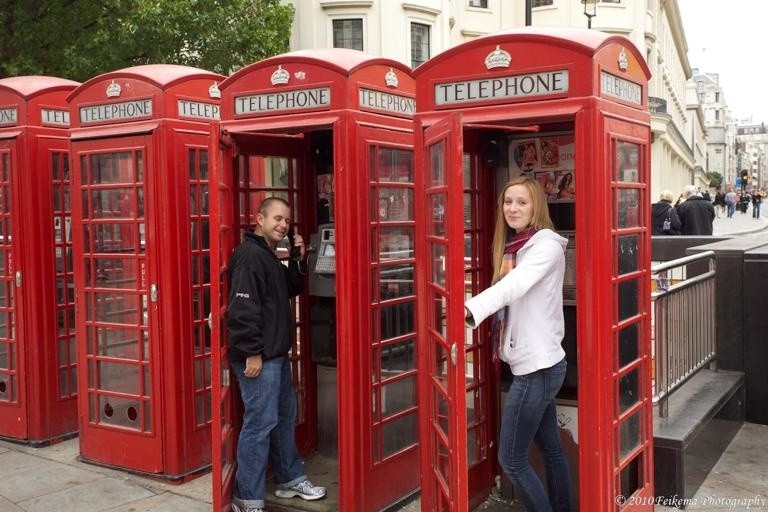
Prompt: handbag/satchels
<box><xmin>663</xmin><ymin>218</ymin><xmax>679</xmax><ymax>235</ymax></box>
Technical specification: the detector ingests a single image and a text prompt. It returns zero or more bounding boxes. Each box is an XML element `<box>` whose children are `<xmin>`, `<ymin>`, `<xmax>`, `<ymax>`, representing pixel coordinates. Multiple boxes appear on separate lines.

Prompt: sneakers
<box><xmin>231</xmin><ymin>503</ymin><xmax>263</xmax><ymax>512</ymax></box>
<box><xmin>275</xmin><ymin>479</ymin><xmax>327</xmax><ymax>500</ymax></box>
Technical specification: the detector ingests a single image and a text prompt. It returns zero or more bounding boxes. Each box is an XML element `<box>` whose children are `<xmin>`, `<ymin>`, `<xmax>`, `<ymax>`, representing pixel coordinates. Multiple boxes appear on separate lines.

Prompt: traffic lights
<box><xmin>741</xmin><ymin>169</ymin><xmax>748</xmax><ymax>185</ymax></box>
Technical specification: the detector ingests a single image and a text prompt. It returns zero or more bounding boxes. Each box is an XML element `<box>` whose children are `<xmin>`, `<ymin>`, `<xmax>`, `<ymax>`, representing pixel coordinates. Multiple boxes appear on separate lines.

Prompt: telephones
<box><xmin>286</xmin><ymin>229</ymin><xmax>300</xmax><ymax>258</ymax></box>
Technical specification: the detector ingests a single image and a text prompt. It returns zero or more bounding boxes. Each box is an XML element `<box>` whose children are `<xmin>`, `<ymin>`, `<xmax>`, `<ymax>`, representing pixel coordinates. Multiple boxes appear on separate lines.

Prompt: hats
<box><xmin>661</xmin><ymin>194</ymin><xmax>672</xmax><ymax>202</ymax></box>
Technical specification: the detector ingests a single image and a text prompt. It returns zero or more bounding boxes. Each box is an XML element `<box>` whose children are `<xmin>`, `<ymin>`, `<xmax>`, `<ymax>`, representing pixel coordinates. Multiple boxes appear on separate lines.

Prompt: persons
<box><xmin>463</xmin><ymin>175</ymin><xmax>571</xmax><ymax>512</ymax></box>
<box><xmin>523</xmin><ymin>140</ymin><xmax>575</xmax><ymax>199</ymax></box>
<box><xmin>651</xmin><ymin>184</ymin><xmax>766</xmax><ymax>291</ymax></box>
<box><xmin>227</xmin><ymin>197</ymin><xmax>327</xmax><ymax>512</ymax></box>
